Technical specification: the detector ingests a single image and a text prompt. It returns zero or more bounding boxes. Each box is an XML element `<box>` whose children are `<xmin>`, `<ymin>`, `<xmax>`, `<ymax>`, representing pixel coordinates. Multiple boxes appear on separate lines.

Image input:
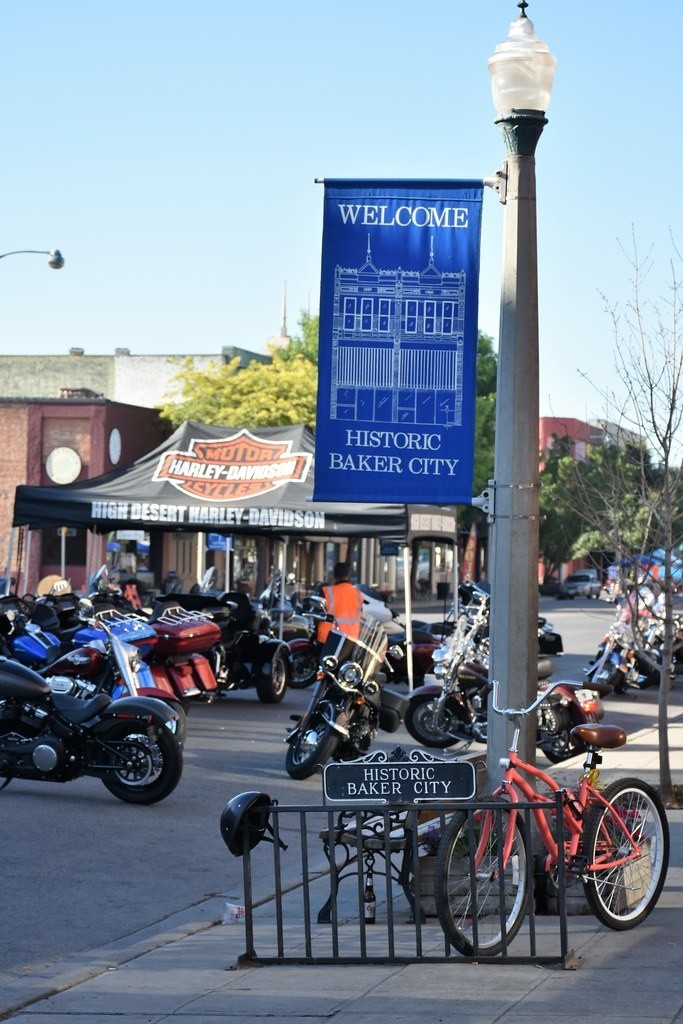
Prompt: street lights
<box><xmin>482</xmin><ymin>0</ymin><xmax>559</xmax><ymax>858</ymax></box>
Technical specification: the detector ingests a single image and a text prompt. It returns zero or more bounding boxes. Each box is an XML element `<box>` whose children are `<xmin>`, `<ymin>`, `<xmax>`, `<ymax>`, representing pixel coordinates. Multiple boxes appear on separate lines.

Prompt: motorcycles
<box><xmin>0</xmin><ymin>547</ymin><xmax>683</xmax><ymax>809</ymax></box>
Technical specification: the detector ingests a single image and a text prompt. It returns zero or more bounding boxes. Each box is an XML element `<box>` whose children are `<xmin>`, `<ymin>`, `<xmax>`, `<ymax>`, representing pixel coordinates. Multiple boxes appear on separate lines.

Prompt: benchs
<box><xmin>316</xmin><ymin>751</ymin><xmax>486</xmax><ymax>925</ymax></box>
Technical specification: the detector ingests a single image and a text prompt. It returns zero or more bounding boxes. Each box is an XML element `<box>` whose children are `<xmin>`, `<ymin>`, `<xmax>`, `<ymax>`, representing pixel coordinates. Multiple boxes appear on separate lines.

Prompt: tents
<box><xmin>3</xmin><ymin>422</ymin><xmax>460</xmax><ymax>694</ymax></box>
<box><xmin>608</xmin><ymin>548</ymin><xmax>683</xmax><ymax>583</ymax></box>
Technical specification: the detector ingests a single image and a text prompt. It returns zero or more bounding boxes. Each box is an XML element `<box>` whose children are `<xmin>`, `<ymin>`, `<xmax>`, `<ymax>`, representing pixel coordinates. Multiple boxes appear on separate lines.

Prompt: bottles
<box><xmin>364</xmin><ymin>873</ymin><xmax>376</xmax><ymax>924</ymax></box>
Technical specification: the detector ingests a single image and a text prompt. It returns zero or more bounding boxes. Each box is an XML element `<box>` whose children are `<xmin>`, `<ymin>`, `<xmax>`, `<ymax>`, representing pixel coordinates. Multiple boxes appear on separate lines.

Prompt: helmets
<box><xmin>36</xmin><ymin>575</ymin><xmax>71</xmax><ymax>597</ymax></box>
<box><xmin>220</xmin><ymin>791</ymin><xmax>271</xmax><ymax>857</ymax></box>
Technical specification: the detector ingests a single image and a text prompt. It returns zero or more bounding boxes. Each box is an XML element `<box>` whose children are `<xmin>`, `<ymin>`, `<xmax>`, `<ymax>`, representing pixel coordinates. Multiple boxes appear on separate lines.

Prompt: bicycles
<box><xmin>433</xmin><ymin>679</ymin><xmax>672</xmax><ymax>961</ymax></box>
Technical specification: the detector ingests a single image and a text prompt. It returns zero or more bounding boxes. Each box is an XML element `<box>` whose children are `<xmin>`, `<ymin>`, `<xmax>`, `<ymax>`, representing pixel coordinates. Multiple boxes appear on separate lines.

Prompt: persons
<box><xmin>120</xmin><ymin>539</ymin><xmax>137</xmax><ymax>576</ymax></box>
<box><xmin>312</xmin><ymin>563</ymin><xmax>364</xmax><ymax>655</ymax></box>
